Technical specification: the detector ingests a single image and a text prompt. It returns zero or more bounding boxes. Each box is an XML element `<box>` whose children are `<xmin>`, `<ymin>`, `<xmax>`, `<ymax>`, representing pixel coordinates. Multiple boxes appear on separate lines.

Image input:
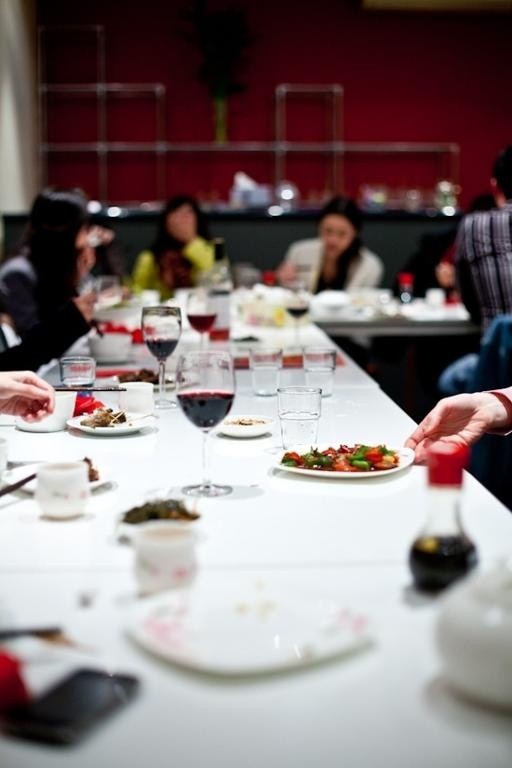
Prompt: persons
<box><xmin>127</xmin><ymin>192</ymin><xmax>214</xmax><ymax>291</ymax></box>
<box><xmin>272</xmin><ymin>193</ymin><xmax>386</xmax><ymax>368</ymax></box>
<box><xmin>0</xmin><ymin>178</ymin><xmax>111</xmax><ymax>424</ymax></box>
<box><xmin>390</xmin><ymin>142</ymin><xmax>511</xmax><ymax>508</ymax></box>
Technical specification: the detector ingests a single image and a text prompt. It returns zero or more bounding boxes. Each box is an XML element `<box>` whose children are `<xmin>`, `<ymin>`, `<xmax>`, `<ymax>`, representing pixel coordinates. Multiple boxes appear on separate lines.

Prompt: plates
<box><xmin>117</xmin><ymin>514</ymin><xmax>206</xmax><ymax>539</ymax></box>
<box><xmin>120</xmin><ymin>562</ymin><xmax>371</xmax><ymax>679</ymax></box>
<box><xmin>61</xmin><ymin>414</ymin><xmax>149</xmax><ymax>441</ymax></box>
<box><xmin>217</xmin><ymin>418</ymin><xmax>271</xmax><ymax>439</ymax></box>
<box><xmin>276</xmin><ymin>453</ymin><xmax>412</xmax><ymax>480</ymax></box>
<box><xmin>111</xmin><ymin>373</ymin><xmax>198</xmax><ymax>394</ymax></box>
<box><xmin>11</xmin><ymin>406</ymin><xmax>69</xmax><ymax>434</ymax></box>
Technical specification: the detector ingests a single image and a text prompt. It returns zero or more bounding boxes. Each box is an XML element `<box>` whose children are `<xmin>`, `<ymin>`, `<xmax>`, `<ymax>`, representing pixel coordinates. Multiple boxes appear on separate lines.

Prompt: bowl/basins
<box><xmin>86</xmin><ymin>333</ymin><xmax>133</xmax><ymax>364</ymax></box>
<box><xmin>0</xmin><ymin>464</ymin><xmax>112</xmax><ymax>495</ymax></box>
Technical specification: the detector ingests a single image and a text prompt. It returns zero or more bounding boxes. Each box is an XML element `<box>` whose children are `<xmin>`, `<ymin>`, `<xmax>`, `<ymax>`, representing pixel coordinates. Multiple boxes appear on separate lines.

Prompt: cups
<box><xmin>0</xmin><ymin>435</ymin><xmax>9</xmax><ymax>478</ymax></box>
<box><xmin>32</xmin><ymin>461</ymin><xmax>91</xmax><ymax>521</ymax></box>
<box><xmin>116</xmin><ymin>379</ymin><xmax>155</xmax><ymax>415</ymax></box>
<box><xmin>274</xmin><ymin>385</ymin><xmax>327</xmax><ymax>454</ymax></box>
<box><xmin>58</xmin><ymin>356</ymin><xmax>95</xmax><ymax>398</ymax></box>
<box><xmin>137</xmin><ymin>516</ymin><xmax>196</xmax><ymax>601</ymax></box>
<box><xmin>300</xmin><ymin>346</ymin><xmax>336</xmax><ymax>399</ymax></box>
<box><xmin>75</xmin><ymin>266</ymin><xmax>448</xmax><ymax>326</ymax></box>
<box><xmin>246</xmin><ymin>344</ymin><xmax>288</xmax><ymax>401</ymax></box>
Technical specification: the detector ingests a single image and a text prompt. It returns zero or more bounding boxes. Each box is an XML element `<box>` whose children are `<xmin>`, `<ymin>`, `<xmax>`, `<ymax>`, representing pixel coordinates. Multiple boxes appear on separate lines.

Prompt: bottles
<box><xmin>429</xmin><ymin>542</ymin><xmax>510</xmax><ymax>713</ymax></box>
<box><xmin>405</xmin><ymin>440</ymin><xmax>485</xmax><ymax>591</ymax></box>
<box><xmin>205</xmin><ymin>236</ymin><xmax>229</xmax><ymax>342</ymax></box>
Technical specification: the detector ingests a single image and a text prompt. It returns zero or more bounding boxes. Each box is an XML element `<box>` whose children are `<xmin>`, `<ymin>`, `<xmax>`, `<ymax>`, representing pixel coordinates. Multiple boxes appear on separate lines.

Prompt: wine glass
<box><xmin>186</xmin><ymin>284</ymin><xmax>219</xmax><ymax>355</ymax></box>
<box><xmin>284</xmin><ymin>278</ymin><xmax>315</xmax><ymax>347</ymax></box>
<box><xmin>138</xmin><ymin>306</ymin><xmax>182</xmax><ymax>411</ymax></box>
<box><xmin>171</xmin><ymin>351</ymin><xmax>235</xmax><ymax>500</ymax></box>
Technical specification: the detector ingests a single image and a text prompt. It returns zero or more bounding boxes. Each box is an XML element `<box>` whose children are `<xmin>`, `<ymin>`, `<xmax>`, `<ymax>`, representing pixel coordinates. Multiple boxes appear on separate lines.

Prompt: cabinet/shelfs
<box><xmin>36</xmin><ymin>23</ymin><xmax>459</xmax><ymax>206</ymax></box>
